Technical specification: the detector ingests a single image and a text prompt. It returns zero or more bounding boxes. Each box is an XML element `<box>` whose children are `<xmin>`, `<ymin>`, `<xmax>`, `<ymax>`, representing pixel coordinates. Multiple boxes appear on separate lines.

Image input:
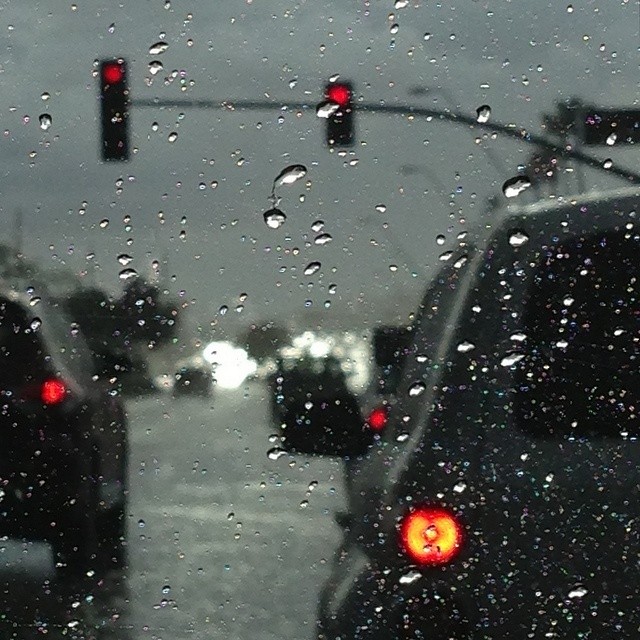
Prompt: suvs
<box><xmin>1</xmin><ymin>277</ymin><xmax>129</xmax><ymax>577</ymax></box>
<box><xmin>271</xmin><ymin>184</ymin><xmax>639</xmax><ymax>640</ymax></box>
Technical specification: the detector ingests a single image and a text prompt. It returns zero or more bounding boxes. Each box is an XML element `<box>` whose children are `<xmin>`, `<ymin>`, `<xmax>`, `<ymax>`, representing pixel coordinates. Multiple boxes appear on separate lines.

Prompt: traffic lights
<box><xmin>95</xmin><ymin>57</ymin><xmax>129</xmax><ymax>160</ymax></box>
<box><xmin>323</xmin><ymin>76</ymin><xmax>357</xmax><ymax>146</ymax></box>
